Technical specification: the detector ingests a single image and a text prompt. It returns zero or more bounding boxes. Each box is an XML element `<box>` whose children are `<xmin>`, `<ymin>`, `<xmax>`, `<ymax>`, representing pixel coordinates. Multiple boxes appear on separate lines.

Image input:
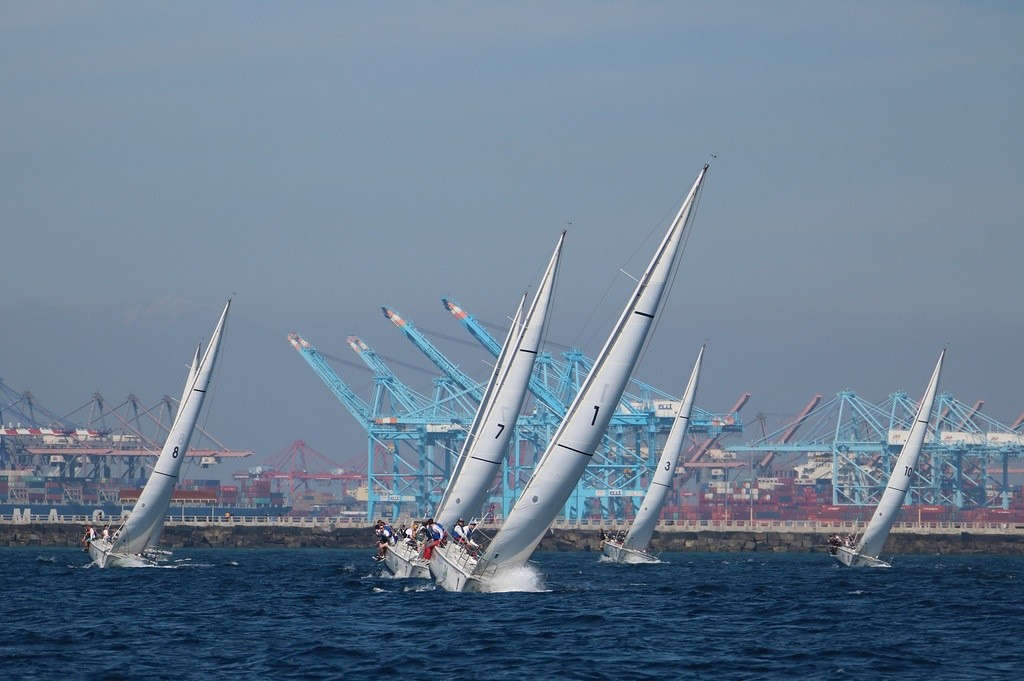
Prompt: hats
<box><xmin>470</xmin><ymin>521</ymin><xmax>476</xmax><ymax>525</ymax></box>
<box><xmin>458</xmin><ymin>518</ymin><xmax>464</xmax><ymax>522</ymax></box>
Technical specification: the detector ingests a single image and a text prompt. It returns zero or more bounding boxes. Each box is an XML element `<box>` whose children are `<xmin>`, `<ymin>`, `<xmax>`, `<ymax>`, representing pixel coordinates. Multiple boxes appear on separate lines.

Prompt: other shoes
<box><xmin>372</xmin><ymin>554</ymin><xmax>380</xmax><ymax>560</ymax></box>
<box><xmin>377</xmin><ymin>557</ymin><xmax>386</xmax><ymax>563</ymax></box>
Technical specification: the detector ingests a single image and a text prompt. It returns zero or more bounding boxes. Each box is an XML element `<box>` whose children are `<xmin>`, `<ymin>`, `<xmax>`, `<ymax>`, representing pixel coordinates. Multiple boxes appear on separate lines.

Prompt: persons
<box><xmin>81</xmin><ymin>524</ymin><xmax>118</xmax><ymax>553</ymax></box>
<box><xmin>225</xmin><ymin>512</ymin><xmax>230</xmax><ymax>522</ymax></box>
<box><xmin>452</xmin><ymin>518</ymin><xmax>479</xmax><ymax>557</ymax></box>
<box><xmin>828</xmin><ymin>533</ymin><xmax>856</xmax><ymax>556</ymax></box>
<box><xmin>598</xmin><ymin>529</ymin><xmax>626</xmax><ymax>551</ymax></box>
<box><xmin>372</xmin><ymin>518</ymin><xmax>447</xmax><ymax>564</ymax></box>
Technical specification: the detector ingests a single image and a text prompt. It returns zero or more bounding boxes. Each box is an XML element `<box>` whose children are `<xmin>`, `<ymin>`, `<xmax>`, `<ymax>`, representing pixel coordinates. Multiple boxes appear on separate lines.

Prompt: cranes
<box><xmin>869</xmin><ymin>399</ymin><xmax>1024</xmax><ymax>487</ymax></box>
<box><xmin>0</xmin><ymin>377</ymin><xmax>254</xmax><ymax>515</ymax></box>
<box><xmin>235</xmin><ymin>297</ymin><xmax>1024</xmax><ymax>523</ymax></box>
<box><xmin>674</xmin><ymin>394</ymin><xmax>823</xmax><ymax>491</ymax></box>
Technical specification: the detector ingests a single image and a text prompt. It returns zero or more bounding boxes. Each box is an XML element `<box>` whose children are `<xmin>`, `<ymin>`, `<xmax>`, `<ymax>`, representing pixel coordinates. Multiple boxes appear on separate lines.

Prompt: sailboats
<box><xmin>836</xmin><ymin>346</ymin><xmax>948</xmax><ymax>569</ymax></box>
<box><xmin>424</xmin><ymin>153</ymin><xmax>718</xmax><ymax>594</ymax></box>
<box><xmin>601</xmin><ymin>343</ymin><xmax>707</xmax><ymax>563</ymax></box>
<box><xmin>379</xmin><ymin>230</ymin><xmax>567</xmax><ymax>577</ymax></box>
<box><xmin>87</xmin><ymin>297</ymin><xmax>233</xmax><ymax>569</ymax></box>
